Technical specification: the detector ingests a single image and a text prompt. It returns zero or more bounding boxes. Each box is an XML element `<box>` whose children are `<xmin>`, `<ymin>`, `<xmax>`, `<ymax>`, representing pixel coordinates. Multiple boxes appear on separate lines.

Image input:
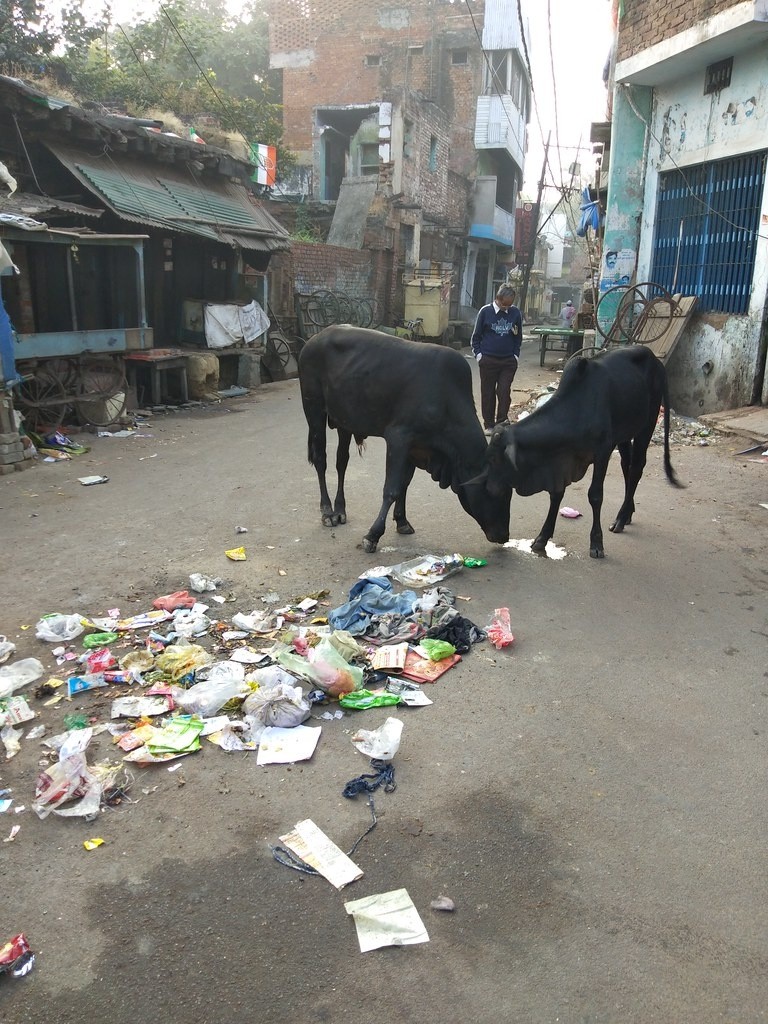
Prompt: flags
<box><xmin>189</xmin><ymin>129</ymin><xmax>277</xmax><ymax>186</ymax></box>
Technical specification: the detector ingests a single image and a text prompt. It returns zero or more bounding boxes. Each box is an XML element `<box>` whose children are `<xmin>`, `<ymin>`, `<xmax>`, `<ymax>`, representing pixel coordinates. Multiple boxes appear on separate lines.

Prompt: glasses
<box><xmin>500</xmin><ymin>300</ymin><xmax>512</xmax><ymax>308</ymax></box>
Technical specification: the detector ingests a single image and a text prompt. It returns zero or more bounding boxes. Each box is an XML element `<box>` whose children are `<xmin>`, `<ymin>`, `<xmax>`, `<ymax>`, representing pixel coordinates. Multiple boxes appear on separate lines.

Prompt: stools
<box><xmin>127</xmin><ymin>355</ymin><xmax>188</xmax><ymax>404</ymax></box>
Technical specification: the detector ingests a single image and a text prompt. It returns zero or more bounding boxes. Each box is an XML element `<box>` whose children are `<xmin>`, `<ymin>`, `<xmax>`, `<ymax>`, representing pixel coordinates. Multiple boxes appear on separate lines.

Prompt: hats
<box><xmin>566</xmin><ymin>300</ymin><xmax>572</xmax><ymax>305</ymax></box>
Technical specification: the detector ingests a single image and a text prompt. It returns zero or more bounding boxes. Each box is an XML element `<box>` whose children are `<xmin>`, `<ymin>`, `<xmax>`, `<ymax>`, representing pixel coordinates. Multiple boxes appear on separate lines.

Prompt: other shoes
<box><xmin>485</xmin><ymin>428</ymin><xmax>494</xmax><ymax>436</ymax></box>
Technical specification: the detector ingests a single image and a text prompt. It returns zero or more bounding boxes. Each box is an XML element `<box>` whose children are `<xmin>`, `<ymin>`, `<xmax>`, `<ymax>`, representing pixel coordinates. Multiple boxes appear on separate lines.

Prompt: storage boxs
<box><xmin>581</xmin><ymin>303</ymin><xmax>594</xmax><ymax>315</ymax></box>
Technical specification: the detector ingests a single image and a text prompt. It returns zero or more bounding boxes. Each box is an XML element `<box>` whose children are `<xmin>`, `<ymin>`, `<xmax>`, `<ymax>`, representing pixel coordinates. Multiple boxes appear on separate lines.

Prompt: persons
<box><xmin>558</xmin><ymin>300</ymin><xmax>575</xmax><ymax>349</ymax></box>
<box><xmin>621</xmin><ymin>275</ymin><xmax>630</xmax><ymax>285</ymax></box>
<box><xmin>605</xmin><ymin>251</ymin><xmax>618</xmax><ymax>270</ymax></box>
<box><xmin>470</xmin><ymin>284</ymin><xmax>522</xmax><ymax>436</ymax></box>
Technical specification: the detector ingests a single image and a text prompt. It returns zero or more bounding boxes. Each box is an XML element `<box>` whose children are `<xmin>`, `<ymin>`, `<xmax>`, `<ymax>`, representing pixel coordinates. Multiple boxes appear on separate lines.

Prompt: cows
<box><xmin>297</xmin><ymin>324</ymin><xmax>686</xmax><ymax>558</ymax></box>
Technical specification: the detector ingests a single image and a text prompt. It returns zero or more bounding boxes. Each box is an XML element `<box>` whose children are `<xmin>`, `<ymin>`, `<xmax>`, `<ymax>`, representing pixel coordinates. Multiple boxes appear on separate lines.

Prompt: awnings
<box><xmin>37</xmin><ymin>134</ymin><xmax>296</xmax><ymax>265</ymax></box>
